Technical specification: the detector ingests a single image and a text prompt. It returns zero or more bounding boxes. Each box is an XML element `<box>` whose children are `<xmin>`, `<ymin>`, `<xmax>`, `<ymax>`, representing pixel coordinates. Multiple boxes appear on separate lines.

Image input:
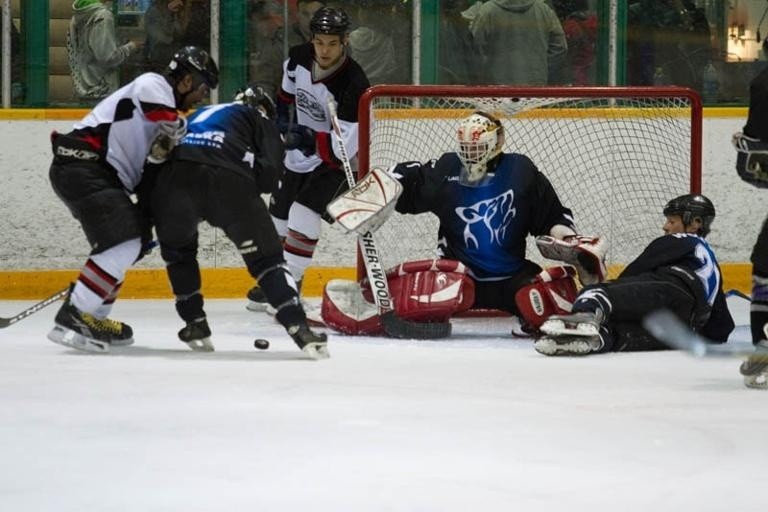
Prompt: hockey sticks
<box><xmin>327</xmin><ymin>103</ymin><xmax>450</xmax><ymax>337</ymax></box>
<box><xmin>0</xmin><ymin>241</ymin><xmax>158</xmax><ymax>328</ymax></box>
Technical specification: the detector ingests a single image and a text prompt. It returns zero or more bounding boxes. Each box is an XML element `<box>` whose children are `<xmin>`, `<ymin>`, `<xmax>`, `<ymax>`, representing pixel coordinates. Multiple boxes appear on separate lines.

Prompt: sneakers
<box><xmin>102</xmin><ymin>318</ymin><xmax>132</xmax><ymax>340</ymax></box>
<box><xmin>741</xmin><ymin>340</ymin><xmax>768</xmax><ymax>375</ymax></box>
<box><xmin>247</xmin><ymin>287</ymin><xmax>268</xmax><ymax>303</ymax></box>
<box><xmin>535</xmin><ymin>336</ymin><xmax>599</xmax><ymax>355</ymax></box>
<box><xmin>288</xmin><ymin>325</ymin><xmax>327</xmax><ymax>348</ymax></box>
<box><xmin>55</xmin><ymin>301</ymin><xmax>105</xmax><ymax>342</ymax></box>
<box><xmin>539</xmin><ymin>312</ymin><xmax>600</xmax><ymax>335</ymax></box>
<box><xmin>178</xmin><ymin>319</ymin><xmax>210</xmax><ymax>341</ymax></box>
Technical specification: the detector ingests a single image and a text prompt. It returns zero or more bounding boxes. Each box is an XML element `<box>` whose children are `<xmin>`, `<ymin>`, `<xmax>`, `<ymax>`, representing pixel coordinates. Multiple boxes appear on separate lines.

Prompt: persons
<box><xmin>322</xmin><ymin>111</ymin><xmax>607</xmax><ymax>339</ymax></box>
<box><xmin>533</xmin><ymin>194</ymin><xmax>735</xmax><ymax>356</ymax></box>
<box><xmin>245</xmin><ymin>6</ymin><xmax>371</xmax><ymax>314</ymax></box>
<box><xmin>149</xmin><ymin>85</ymin><xmax>331</xmax><ymax>360</ymax></box>
<box><xmin>49</xmin><ymin>46</ymin><xmax>218</xmax><ymax>354</ymax></box>
<box><xmin>65</xmin><ymin>0</ymin><xmax>145</xmax><ymax>107</ymax></box>
<box><xmin>247</xmin><ymin>2</ymin><xmax>715</xmax><ymax>107</ymax></box>
<box><xmin>732</xmin><ymin>36</ymin><xmax>768</xmax><ymax>389</ymax></box>
<box><xmin>142</xmin><ymin>0</ymin><xmax>210</xmax><ymax>74</ymax></box>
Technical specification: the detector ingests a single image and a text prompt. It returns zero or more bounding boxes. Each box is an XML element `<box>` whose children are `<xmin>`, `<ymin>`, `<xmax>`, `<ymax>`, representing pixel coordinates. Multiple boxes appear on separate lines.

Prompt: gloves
<box><xmin>732</xmin><ymin>134</ymin><xmax>767</xmax><ymax>187</ymax></box>
<box><xmin>285</xmin><ymin>125</ymin><xmax>315</xmax><ymax>155</ymax></box>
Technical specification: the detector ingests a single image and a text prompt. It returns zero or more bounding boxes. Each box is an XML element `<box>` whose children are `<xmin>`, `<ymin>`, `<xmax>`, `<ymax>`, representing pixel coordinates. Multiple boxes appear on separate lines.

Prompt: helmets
<box><xmin>311</xmin><ymin>7</ymin><xmax>349</xmax><ymax>35</ymax></box>
<box><xmin>664</xmin><ymin>194</ymin><xmax>714</xmax><ymax>233</ymax></box>
<box><xmin>235</xmin><ymin>85</ymin><xmax>276</xmax><ymax>116</ymax></box>
<box><xmin>168</xmin><ymin>46</ymin><xmax>218</xmax><ymax>91</ymax></box>
<box><xmin>456</xmin><ymin>112</ymin><xmax>505</xmax><ymax>174</ymax></box>
